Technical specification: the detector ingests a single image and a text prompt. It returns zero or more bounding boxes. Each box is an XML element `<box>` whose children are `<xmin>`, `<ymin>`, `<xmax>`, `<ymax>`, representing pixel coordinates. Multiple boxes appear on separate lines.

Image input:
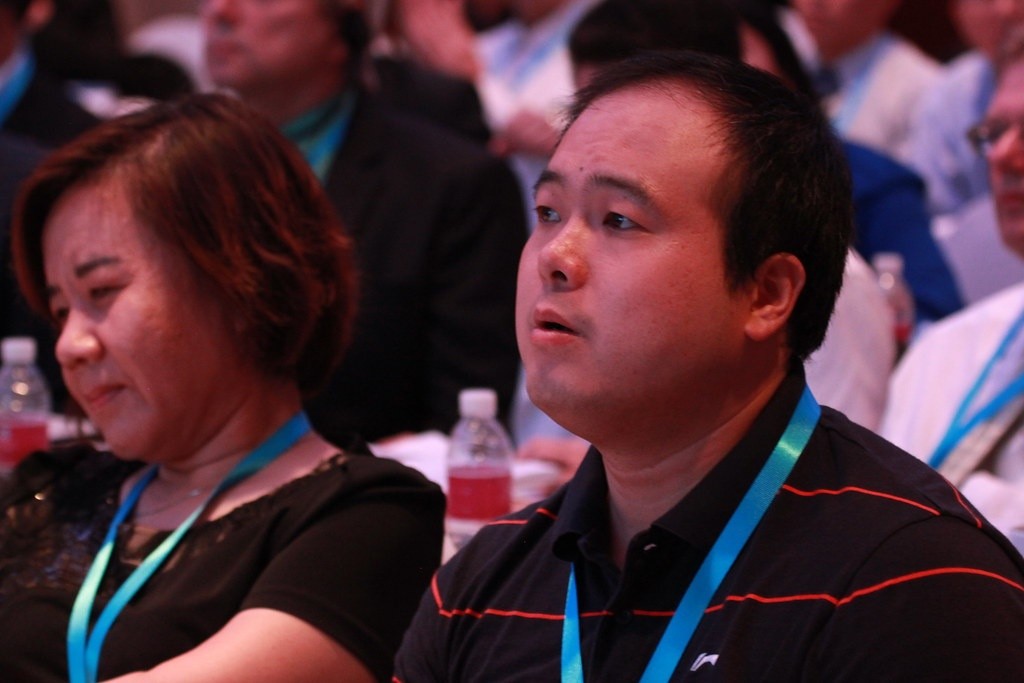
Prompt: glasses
<box><xmin>968</xmin><ymin>121</ymin><xmax>1008</xmax><ymax>159</ymax></box>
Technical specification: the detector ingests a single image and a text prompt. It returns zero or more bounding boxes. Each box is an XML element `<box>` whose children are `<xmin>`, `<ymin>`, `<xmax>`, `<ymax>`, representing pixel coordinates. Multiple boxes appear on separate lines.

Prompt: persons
<box><xmin>0</xmin><ymin>95</ymin><xmax>443</xmax><ymax>683</ymax></box>
<box><xmin>392</xmin><ymin>47</ymin><xmax>1024</xmax><ymax>683</ymax></box>
<box><xmin>0</xmin><ymin>0</ymin><xmax>1024</xmax><ymax>554</ymax></box>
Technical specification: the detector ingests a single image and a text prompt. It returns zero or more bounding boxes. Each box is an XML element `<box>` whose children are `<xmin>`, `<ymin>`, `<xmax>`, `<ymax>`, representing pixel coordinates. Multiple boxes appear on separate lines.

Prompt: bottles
<box><xmin>872</xmin><ymin>252</ymin><xmax>913</xmax><ymax>363</ymax></box>
<box><xmin>0</xmin><ymin>336</ymin><xmax>49</xmax><ymax>477</ymax></box>
<box><xmin>447</xmin><ymin>388</ymin><xmax>514</xmax><ymax>558</ymax></box>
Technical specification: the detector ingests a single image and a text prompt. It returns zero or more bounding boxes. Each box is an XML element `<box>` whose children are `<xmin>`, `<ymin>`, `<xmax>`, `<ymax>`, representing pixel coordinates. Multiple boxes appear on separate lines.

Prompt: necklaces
<box><xmin>131</xmin><ymin>434</ymin><xmax>321</xmax><ymax>519</ymax></box>
